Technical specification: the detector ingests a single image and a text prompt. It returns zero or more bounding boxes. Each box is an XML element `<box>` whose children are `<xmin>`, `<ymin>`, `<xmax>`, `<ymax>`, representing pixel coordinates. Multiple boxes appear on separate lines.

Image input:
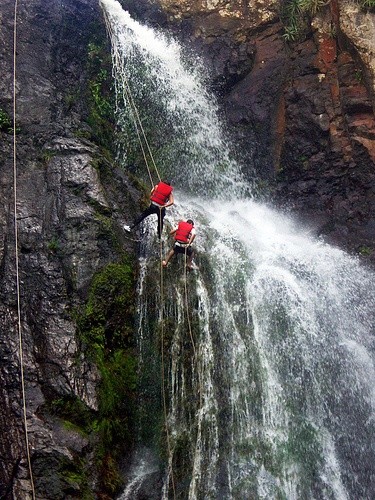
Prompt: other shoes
<box><xmin>186</xmin><ymin>264</ymin><xmax>195</xmax><ymax>270</ymax></box>
<box><xmin>162</xmin><ymin>260</ymin><xmax>166</xmax><ymax>268</ymax></box>
<box><xmin>124</xmin><ymin>225</ymin><xmax>130</xmax><ymax>232</ymax></box>
<box><xmin>156</xmin><ymin>238</ymin><xmax>164</xmax><ymax>243</ymax></box>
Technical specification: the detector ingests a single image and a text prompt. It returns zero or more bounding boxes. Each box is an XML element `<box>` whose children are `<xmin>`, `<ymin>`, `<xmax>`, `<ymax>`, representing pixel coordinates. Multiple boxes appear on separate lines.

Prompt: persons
<box><xmin>124</xmin><ymin>180</ymin><xmax>174</xmax><ymax>244</ymax></box>
<box><xmin>161</xmin><ymin>219</ymin><xmax>197</xmax><ymax>271</ymax></box>
